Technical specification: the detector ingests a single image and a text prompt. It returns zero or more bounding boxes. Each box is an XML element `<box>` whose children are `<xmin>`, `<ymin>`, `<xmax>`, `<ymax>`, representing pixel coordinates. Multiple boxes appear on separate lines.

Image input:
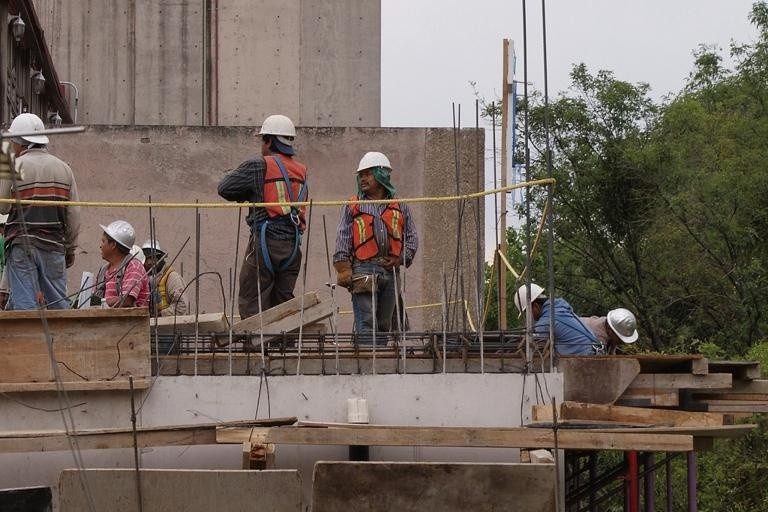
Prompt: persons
<box><xmin>0</xmin><ymin>113</ymin><xmax>79</xmax><ymax>310</ymax></box>
<box><xmin>92</xmin><ymin>220</ymin><xmax>188</xmax><ymax>316</ymax></box>
<box><xmin>215</xmin><ymin>113</ymin><xmax>309</xmax><ymax>327</ymax></box>
<box><xmin>331</xmin><ymin>149</ymin><xmax>418</xmax><ymax>349</ymax></box>
<box><xmin>571</xmin><ymin>307</ymin><xmax>638</xmax><ymax>356</ymax></box>
<box><xmin>512</xmin><ymin>284</ymin><xmax>605</xmax><ymax>362</ymax></box>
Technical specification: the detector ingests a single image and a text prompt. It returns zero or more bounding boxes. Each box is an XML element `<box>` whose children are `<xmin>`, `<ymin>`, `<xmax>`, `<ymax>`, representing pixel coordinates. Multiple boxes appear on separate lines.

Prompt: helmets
<box><xmin>8</xmin><ymin>113</ymin><xmax>50</xmax><ymax>144</ymax></box>
<box><xmin>607</xmin><ymin>308</ymin><xmax>639</xmax><ymax>344</ymax></box>
<box><xmin>514</xmin><ymin>283</ymin><xmax>545</xmax><ymax>319</ymax></box>
<box><xmin>98</xmin><ymin>221</ymin><xmax>167</xmax><ymax>265</ymax></box>
<box><xmin>357</xmin><ymin>151</ymin><xmax>392</xmax><ymax>173</ymax></box>
<box><xmin>259</xmin><ymin>115</ymin><xmax>297</xmax><ymax>137</ymax></box>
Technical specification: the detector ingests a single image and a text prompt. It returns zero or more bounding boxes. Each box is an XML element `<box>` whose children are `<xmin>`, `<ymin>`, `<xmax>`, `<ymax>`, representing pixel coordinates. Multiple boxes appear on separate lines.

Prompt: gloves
<box><xmin>333</xmin><ymin>261</ymin><xmax>352</xmax><ymax>288</ymax></box>
